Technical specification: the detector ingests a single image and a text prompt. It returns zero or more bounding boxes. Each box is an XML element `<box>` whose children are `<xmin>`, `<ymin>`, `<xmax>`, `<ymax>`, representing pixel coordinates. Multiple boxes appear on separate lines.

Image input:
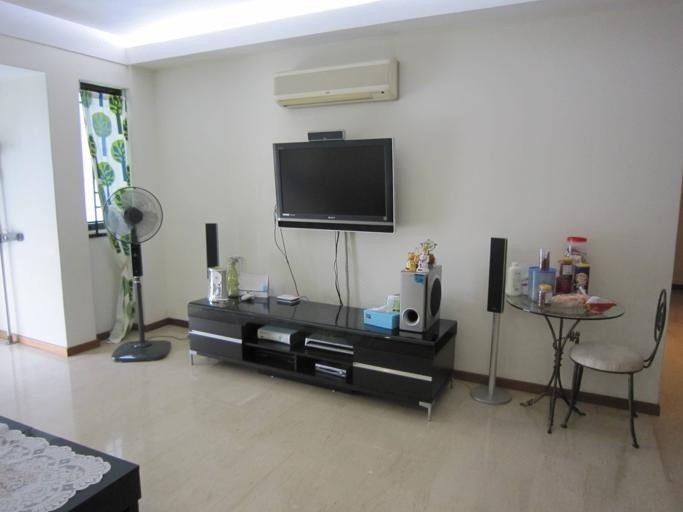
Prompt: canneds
<box><xmin>538</xmin><ymin>284</ymin><xmax>553</xmax><ymax>308</ymax></box>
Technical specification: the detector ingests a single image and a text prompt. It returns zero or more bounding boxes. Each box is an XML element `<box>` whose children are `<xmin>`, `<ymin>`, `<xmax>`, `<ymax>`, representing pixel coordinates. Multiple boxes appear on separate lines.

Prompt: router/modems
<box><xmin>277</xmin><ymin>295</ymin><xmax>300</xmax><ymax>305</ymax></box>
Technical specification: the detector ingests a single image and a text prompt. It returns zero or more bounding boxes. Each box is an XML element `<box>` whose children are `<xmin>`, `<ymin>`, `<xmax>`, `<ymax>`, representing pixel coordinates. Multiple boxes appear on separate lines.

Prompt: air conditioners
<box><xmin>273</xmin><ymin>58</ymin><xmax>399</xmax><ymax>110</ymax></box>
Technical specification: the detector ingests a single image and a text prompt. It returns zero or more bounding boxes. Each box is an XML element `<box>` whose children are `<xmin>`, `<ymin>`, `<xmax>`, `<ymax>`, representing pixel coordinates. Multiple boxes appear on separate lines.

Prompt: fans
<box><xmin>102</xmin><ymin>187</ymin><xmax>171</xmax><ymax>361</ymax></box>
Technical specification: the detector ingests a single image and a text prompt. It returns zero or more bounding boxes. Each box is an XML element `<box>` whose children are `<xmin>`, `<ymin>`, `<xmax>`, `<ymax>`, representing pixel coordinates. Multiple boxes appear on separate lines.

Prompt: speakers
<box><xmin>486</xmin><ymin>238</ymin><xmax>508</xmax><ymax>314</ymax></box>
<box><xmin>399</xmin><ymin>264</ymin><xmax>442</xmax><ymax>334</ymax></box>
<box><xmin>205</xmin><ymin>223</ymin><xmax>219</xmax><ymax>277</ymax></box>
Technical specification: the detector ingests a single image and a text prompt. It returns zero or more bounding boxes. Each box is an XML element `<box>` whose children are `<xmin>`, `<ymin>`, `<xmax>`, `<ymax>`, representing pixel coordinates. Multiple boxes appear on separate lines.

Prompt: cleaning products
<box><xmin>225</xmin><ymin>255</ymin><xmax>240</xmax><ymax>297</ymax></box>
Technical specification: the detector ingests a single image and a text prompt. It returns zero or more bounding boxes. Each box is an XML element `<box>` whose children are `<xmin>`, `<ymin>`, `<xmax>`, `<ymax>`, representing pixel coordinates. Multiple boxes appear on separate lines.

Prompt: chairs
<box><xmin>561</xmin><ymin>288</ymin><xmax>666</xmax><ymax>448</ymax></box>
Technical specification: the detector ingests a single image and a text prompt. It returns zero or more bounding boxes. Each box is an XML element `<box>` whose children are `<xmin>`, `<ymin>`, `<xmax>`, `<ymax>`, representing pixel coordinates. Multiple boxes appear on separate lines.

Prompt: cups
<box><xmin>537</xmin><ymin>248</ymin><xmax>550</xmax><ymax>272</ymax></box>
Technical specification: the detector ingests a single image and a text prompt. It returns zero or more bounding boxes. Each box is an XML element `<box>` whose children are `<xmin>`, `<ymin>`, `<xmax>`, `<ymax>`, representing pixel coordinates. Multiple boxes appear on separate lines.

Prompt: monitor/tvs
<box><xmin>272</xmin><ymin>137</ymin><xmax>394</xmax><ymax>234</ymax></box>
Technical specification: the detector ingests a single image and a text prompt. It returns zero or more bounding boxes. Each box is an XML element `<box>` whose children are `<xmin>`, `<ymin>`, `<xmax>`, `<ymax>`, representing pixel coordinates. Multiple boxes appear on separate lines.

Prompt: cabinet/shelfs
<box><xmin>187</xmin><ymin>289</ymin><xmax>457</xmax><ymax>421</ymax></box>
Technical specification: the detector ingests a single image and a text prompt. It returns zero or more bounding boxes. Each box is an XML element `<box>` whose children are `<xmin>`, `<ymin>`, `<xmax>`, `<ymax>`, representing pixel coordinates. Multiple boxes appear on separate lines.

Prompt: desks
<box><xmin>508</xmin><ymin>293</ymin><xmax>625</xmax><ymax>434</ymax></box>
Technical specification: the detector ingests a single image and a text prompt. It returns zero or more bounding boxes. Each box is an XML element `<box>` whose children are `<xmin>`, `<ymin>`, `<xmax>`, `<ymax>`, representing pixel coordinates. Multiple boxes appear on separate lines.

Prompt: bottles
<box><xmin>558</xmin><ymin>236</ymin><xmax>589</xmax><ymax>292</ymax></box>
<box><xmin>536</xmin><ymin>285</ymin><xmax>552</xmax><ymax>306</ymax></box>
<box><xmin>506</xmin><ymin>260</ymin><xmax>523</xmax><ymax>294</ymax></box>
<box><xmin>406</xmin><ymin>251</ymin><xmax>429</xmax><ymax>273</ymax></box>
